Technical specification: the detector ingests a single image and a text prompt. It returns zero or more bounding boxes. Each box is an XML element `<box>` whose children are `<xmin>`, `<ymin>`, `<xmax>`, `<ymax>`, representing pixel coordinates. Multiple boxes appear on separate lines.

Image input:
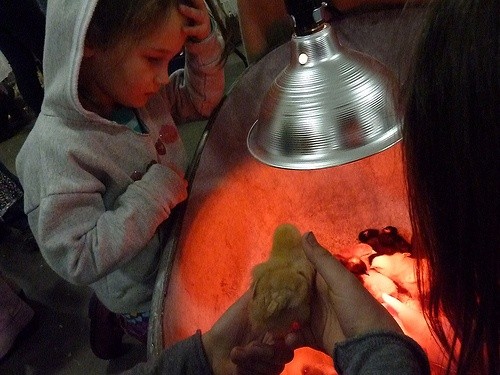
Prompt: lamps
<box><xmin>246</xmin><ymin>2</ymin><xmax>429</xmax><ymax>175</ymax></box>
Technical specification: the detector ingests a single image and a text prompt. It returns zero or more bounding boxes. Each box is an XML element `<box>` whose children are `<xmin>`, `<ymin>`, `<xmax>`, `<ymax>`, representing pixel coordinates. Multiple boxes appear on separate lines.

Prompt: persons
<box><xmin>0</xmin><ymin>0</ymin><xmax>46</xmax><ymax>120</ymax></box>
<box><xmin>13</xmin><ymin>0</ymin><xmax>225</xmax><ymax>360</ymax></box>
<box><xmin>114</xmin><ymin>0</ymin><xmax>500</xmax><ymax>375</ymax></box>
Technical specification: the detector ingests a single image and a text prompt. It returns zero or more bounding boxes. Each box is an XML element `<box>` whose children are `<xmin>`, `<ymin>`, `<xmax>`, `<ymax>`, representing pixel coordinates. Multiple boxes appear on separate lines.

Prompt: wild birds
<box><xmin>333</xmin><ymin>225</ymin><xmax>438</xmax><ymax>349</ymax></box>
<box><xmin>246</xmin><ymin>223</ymin><xmax>316</xmax><ymax>346</ymax></box>
